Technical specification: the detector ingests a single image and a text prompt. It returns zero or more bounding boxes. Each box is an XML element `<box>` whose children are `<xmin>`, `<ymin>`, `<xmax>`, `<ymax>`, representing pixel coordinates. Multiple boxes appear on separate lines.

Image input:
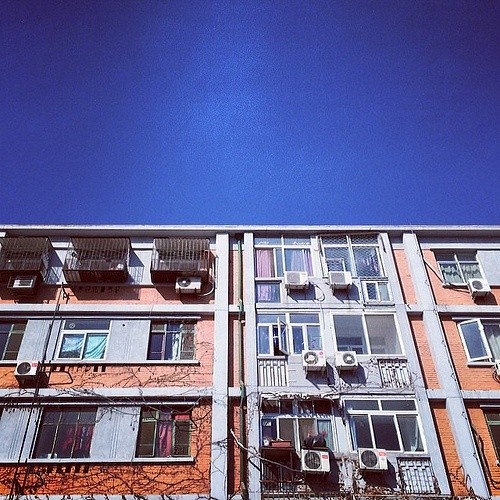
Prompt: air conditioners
<box><xmin>300</xmin><ymin>449</ymin><xmax>331</xmax><ymax>475</ymax></box>
<box><xmin>13</xmin><ymin>275</ymin><xmax>36</xmax><ymax>294</ymax></box>
<box><xmin>283</xmin><ymin>270</ymin><xmax>309</xmax><ymax>290</ymax></box>
<box><xmin>301</xmin><ymin>349</ymin><xmax>326</xmax><ymax>371</ymax></box>
<box><xmin>334</xmin><ymin>350</ymin><xmax>358</xmax><ymax>371</ymax></box>
<box><xmin>175</xmin><ymin>276</ymin><xmax>202</xmax><ymax>294</ymax></box>
<box><xmin>328</xmin><ymin>271</ymin><xmax>353</xmax><ymax>289</ymax></box>
<box><xmin>494</xmin><ymin>358</ymin><xmax>500</xmax><ymax>378</ymax></box>
<box><xmin>357</xmin><ymin>448</ymin><xmax>388</xmax><ymax>473</ymax></box>
<box><xmin>466</xmin><ymin>277</ymin><xmax>491</xmax><ymax>297</ymax></box>
<box><xmin>12</xmin><ymin>360</ymin><xmax>41</xmax><ymax>382</ymax></box>
